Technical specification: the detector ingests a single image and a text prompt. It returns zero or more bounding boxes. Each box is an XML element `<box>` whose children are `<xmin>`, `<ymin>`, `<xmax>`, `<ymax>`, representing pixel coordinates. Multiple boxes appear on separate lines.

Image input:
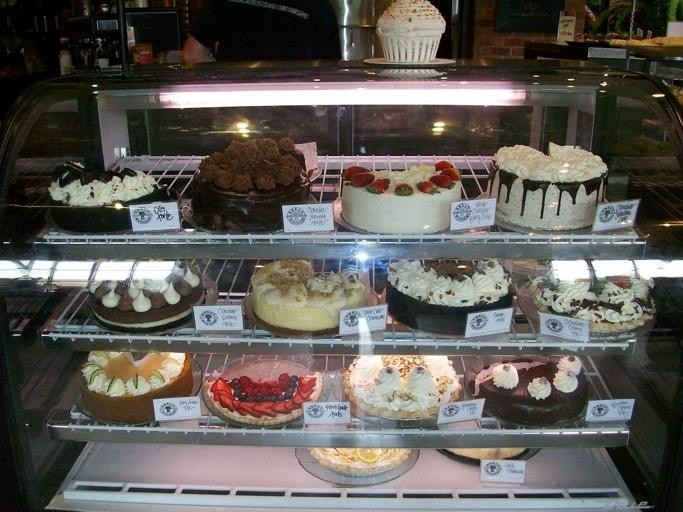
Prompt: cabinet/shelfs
<box><xmin>1</xmin><ymin>0</ymin><xmax>200</xmax><ymax>88</ymax></box>
<box><xmin>0</xmin><ymin>67</ymin><xmax>682</xmax><ymax>511</ymax></box>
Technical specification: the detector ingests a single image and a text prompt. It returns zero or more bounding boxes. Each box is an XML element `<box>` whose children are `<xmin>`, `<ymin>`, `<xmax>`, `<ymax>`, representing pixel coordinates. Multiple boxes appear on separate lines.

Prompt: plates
<box><xmin>364</xmin><ymin>57</ymin><xmax>456</xmax><ymax>65</ymax></box>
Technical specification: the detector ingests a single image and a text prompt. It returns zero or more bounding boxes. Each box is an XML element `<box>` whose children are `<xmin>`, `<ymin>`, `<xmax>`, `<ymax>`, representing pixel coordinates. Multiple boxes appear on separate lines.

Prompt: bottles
<box><xmin>58</xmin><ymin>36</ymin><xmax>105</xmax><ymax>76</ymax></box>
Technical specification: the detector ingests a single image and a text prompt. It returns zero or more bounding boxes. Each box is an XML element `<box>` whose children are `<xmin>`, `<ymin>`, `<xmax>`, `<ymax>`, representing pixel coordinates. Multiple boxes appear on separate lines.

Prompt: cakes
<box><xmin>77</xmin><ymin>351</ymin><xmax>193</xmax><ymax>423</ymax></box>
<box><xmin>340</xmin><ymin>160</ymin><xmax>462</xmax><ymax>234</ymax></box>
<box><xmin>386</xmin><ymin>258</ymin><xmax>513</xmax><ymax>334</ymax></box>
<box><xmin>375</xmin><ymin>0</ymin><xmax>447</xmax><ymax>64</ymax></box>
<box><xmin>471</xmin><ymin>354</ymin><xmax>589</xmax><ymax>425</ymax></box>
<box><xmin>249</xmin><ymin>259</ymin><xmax>378</xmax><ymax>332</ymax></box>
<box><xmin>528</xmin><ymin>262</ymin><xmax>655</xmax><ymax>334</ymax></box>
<box><xmin>307</xmin><ymin>448</ymin><xmax>414</xmax><ymax>476</ymax></box>
<box><xmin>46</xmin><ymin>157</ymin><xmax>171</xmax><ymax>232</ymax></box>
<box><xmin>204</xmin><ymin>370</ymin><xmax>324</xmax><ymax>426</ymax></box>
<box><xmin>445</xmin><ymin>447</ymin><xmax>529</xmax><ymax>460</ymax></box>
<box><xmin>342</xmin><ymin>355</ymin><xmax>463</xmax><ymax>421</ymax></box>
<box><xmin>87</xmin><ymin>259</ymin><xmax>205</xmax><ymax>332</ymax></box>
<box><xmin>190</xmin><ymin>136</ymin><xmax>311</xmax><ymax>234</ymax></box>
<box><xmin>487</xmin><ymin>141</ymin><xmax>609</xmax><ymax>231</ymax></box>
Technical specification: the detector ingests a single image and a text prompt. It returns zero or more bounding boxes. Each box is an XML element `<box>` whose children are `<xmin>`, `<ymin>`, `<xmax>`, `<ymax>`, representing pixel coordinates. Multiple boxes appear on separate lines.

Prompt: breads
<box><xmin>608</xmin><ymin>36</ymin><xmax>683</xmax><ymax>47</ymax></box>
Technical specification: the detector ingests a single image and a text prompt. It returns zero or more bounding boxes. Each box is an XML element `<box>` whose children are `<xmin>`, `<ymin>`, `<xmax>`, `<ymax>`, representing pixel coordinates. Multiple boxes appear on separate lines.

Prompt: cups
<box><xmin>96</xmin><ymin>58</ymin><xmax>110</xmax><ymax>69</ymax></box>
